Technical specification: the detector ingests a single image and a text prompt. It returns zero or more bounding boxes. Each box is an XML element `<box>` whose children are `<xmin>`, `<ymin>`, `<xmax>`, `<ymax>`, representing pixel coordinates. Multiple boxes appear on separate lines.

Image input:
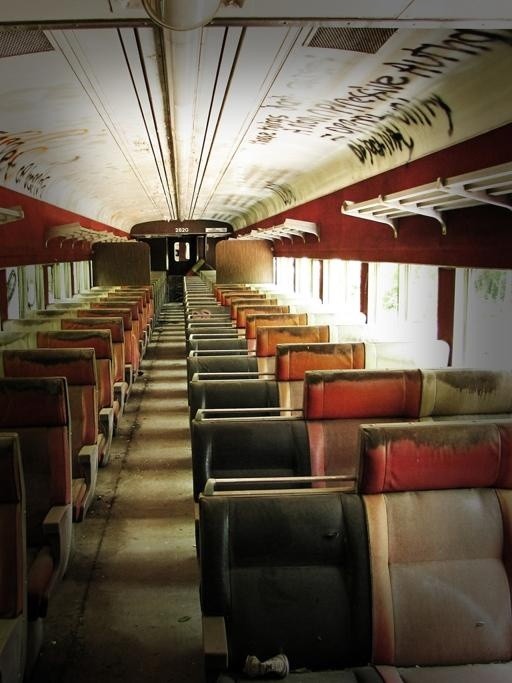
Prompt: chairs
<box><xmin>0</xmin><ymin>270</ymin><xmax>166</xmax><ymax>683</ymax></box>
<box><xmin>184</xmin><ymin>271</ymin><xmax>512</xmax><ymax>683</ymax></box>
